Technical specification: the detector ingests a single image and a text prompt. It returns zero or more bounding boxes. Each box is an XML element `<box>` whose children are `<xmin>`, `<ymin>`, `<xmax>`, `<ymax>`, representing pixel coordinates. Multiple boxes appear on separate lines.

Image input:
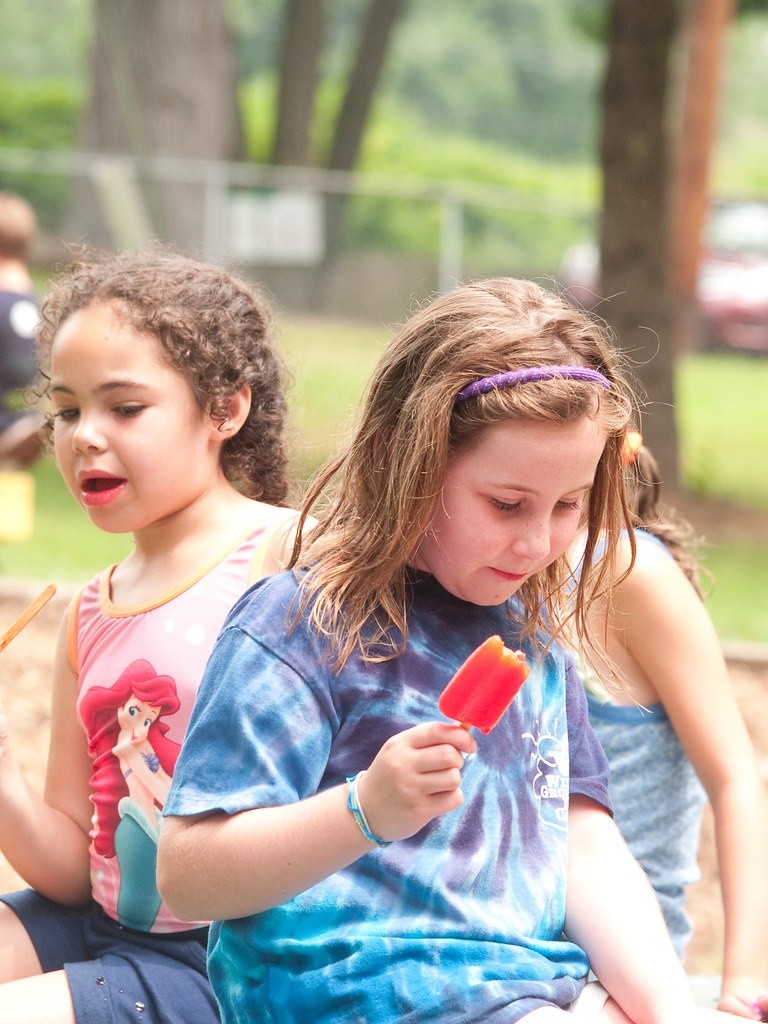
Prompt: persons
<box><xmin>0</xmin><ymin>193</ymin><xmax>52</xmax><ymax>539</ymax></box>
<box><xmin>0</xmin><ymin>250</ymin><xmax>321</xmax><ymax>1024</ymax></box>
<box><xmin>541</xmin><ymin>431</ymin><xmax>768</xmax><ymax>1020</ymax></box>
<box><xmin>158</xmin><ymin>277</ymin><xmax>768</xmax><ymax>1024</ymax></box>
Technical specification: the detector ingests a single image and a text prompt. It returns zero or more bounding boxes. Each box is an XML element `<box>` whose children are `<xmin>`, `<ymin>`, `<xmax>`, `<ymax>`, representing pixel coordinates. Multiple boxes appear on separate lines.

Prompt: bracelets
<box><xmin>347</xmin><ymin>772</ymin><xmax>390</xmax><ymax>849</ymax></box>
